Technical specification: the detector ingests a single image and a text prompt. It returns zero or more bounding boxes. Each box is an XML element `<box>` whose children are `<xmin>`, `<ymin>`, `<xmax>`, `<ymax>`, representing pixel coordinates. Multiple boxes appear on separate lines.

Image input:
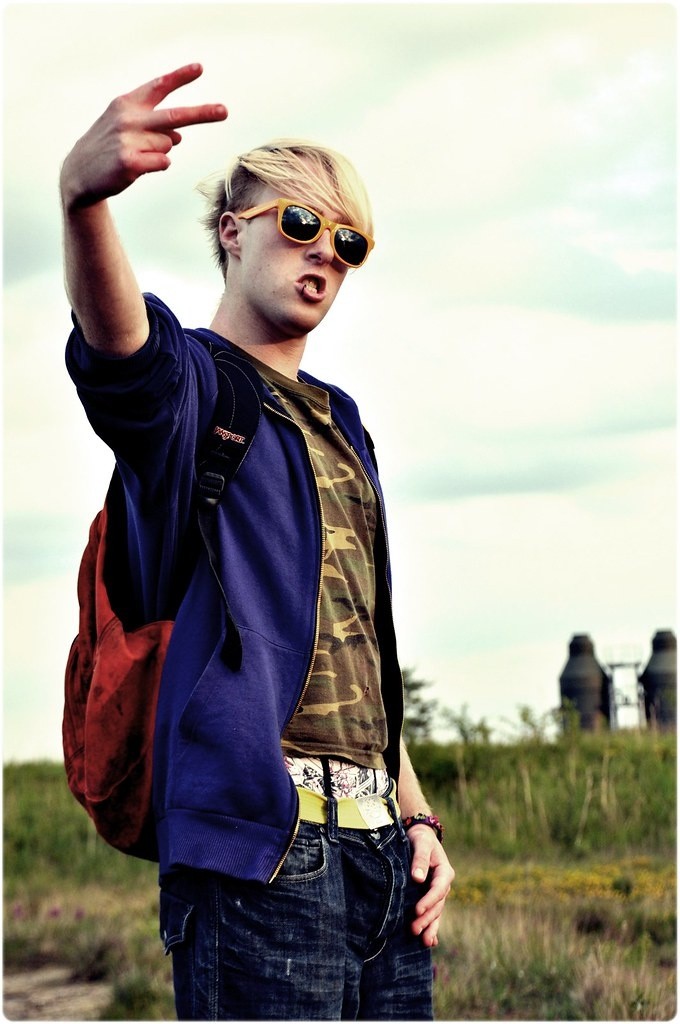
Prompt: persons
<box><xmin>57</xmin><ymin>61</ymin><xmax>455</xmax><ymax>1021</ymax></box>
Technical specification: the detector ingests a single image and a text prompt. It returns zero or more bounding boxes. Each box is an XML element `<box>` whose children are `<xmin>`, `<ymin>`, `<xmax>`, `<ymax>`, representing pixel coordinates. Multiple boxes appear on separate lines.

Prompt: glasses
<box><xmin>236</xmin><ymin>198</ymin><xmax>375</xmax><ymax>269</ymax></box>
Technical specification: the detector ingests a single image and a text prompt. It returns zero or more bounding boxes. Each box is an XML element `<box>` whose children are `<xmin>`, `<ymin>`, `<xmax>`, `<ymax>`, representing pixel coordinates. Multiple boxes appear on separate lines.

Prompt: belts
<box><xmin>294</xmin><ymin>777</ymin><xmax>402</xmax><ymax>830</ymax></box>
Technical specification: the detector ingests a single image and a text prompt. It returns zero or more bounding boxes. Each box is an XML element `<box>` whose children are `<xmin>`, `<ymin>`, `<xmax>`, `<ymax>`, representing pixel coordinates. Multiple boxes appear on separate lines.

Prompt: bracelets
<box><xmin>404</xmin><ymin>812</ymin><xmax>444</xmax><ymax>845</ymax></box>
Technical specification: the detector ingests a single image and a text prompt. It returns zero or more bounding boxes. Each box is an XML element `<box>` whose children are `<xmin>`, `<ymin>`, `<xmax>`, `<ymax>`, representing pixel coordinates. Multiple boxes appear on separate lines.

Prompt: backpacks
<box><xmin>60</xmin><ymin>326</ymin><xmax>264</xmax><ymax>865</ymax></box>
